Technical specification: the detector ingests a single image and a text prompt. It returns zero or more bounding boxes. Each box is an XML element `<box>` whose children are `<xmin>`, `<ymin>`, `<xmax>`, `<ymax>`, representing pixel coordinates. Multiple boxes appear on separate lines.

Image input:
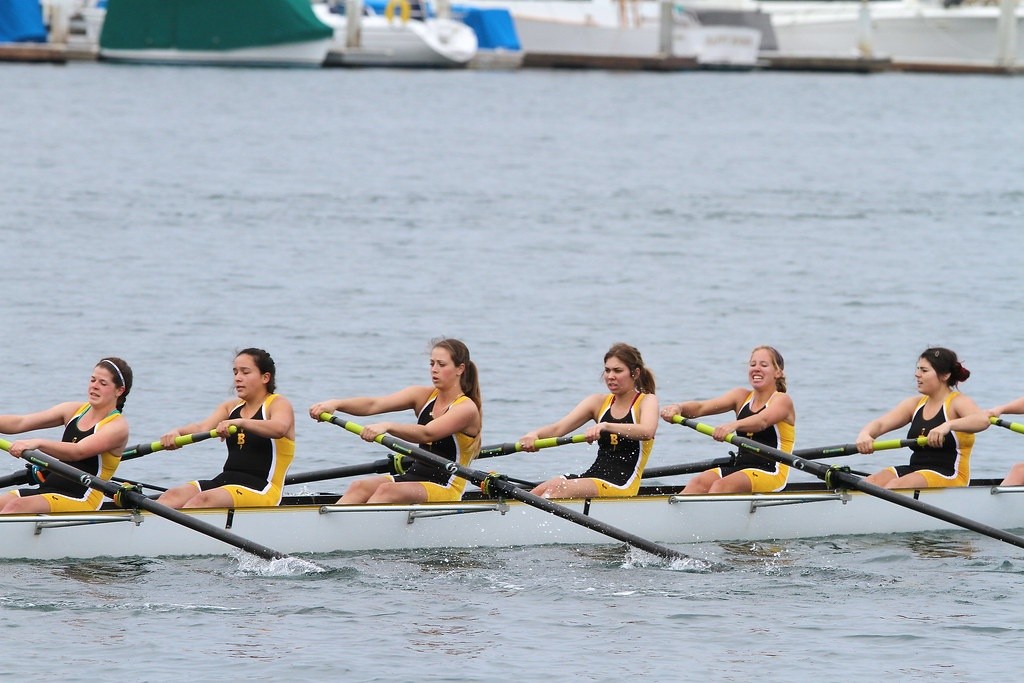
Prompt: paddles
<box><xmin>672</xmin><ymin>413</ymin><xmax>1024</xmax><ymax>550</ymax></box>
<box><xmin>283</xmin><ymin>433</ymin><xmax>601</xmax><ymax>487</ymax></box>
<box><xmin>0</xmin><ymin>424</ymin><xmax>238</xmax><ymax>489</ymax></box>
<box><xmin>0</xmin><ymin>438</ymin><xmax>290</xmax><ymax>562</ymax></box>
<box><xmin>640</xmin><ymin>435</ymin><xmax>946</xmax><ymax>480</ymax></box>
<box><xmin>319</xmin><ymin>411</ymin><xmax>719</xmax><ymax>567</ymax></box>
<box><xmin>989</xmin><ymin>416</ymin><xmax>1024</xmax><ymax>434</ymax></box>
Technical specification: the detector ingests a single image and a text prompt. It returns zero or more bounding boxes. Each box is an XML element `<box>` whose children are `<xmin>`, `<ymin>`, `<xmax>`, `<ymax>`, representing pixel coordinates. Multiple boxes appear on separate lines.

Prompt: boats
<box><xmin>0</xmin><ymin>0</ymin><xmax>1024</xmax><ymax>81</ymax></box>
<box><xmin>0</xmin><ymin>477</ymin><xmax>1024</xmax><ymax>561</ymax></box>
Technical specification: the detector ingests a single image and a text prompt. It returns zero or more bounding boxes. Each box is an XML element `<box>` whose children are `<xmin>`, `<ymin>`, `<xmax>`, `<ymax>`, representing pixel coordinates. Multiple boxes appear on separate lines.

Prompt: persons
<box><xmin>153</xmin><ymin>348</ymin><xmax>295</xmax><ymax>509</ymax></box>
<box><xmin>662</xmin><ymin>345</ymin><xmax>797</xmax><ymax>494</ymax></box>
<box><xmin>308</xmin><ymin>339</ymin><xmax>482</xmax><ymax>504</ymax></box>
<box><xmin>987</xmin><ymin>396</ymin><xmax>1024</xmax><ymax>485</ymax></box>
<box><xmin>520</xmin><ymin>342</ymin><xmax>660</xmax><ymax>499</ymax></box>
<box><xmin>0</xmin><ymin>357</ymin><xmax>133</xmax><ymax>514</ymax></box>
<box><xmin>856</xmin><ymin>348</ymin><xmax>991</xmax><ymax>488</ymax></box>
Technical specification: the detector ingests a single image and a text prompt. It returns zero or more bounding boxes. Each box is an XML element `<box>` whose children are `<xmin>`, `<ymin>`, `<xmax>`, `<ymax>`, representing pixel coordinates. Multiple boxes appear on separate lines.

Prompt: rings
<box><xmin>664</xmin><ymin>410</ymin><xmax>669</xmax><ymax>414</ymax></box>
<box><xmin>522</xmin><ymin>443</ymin><xmax>525</xmax><ymax>448</ymax></box>
